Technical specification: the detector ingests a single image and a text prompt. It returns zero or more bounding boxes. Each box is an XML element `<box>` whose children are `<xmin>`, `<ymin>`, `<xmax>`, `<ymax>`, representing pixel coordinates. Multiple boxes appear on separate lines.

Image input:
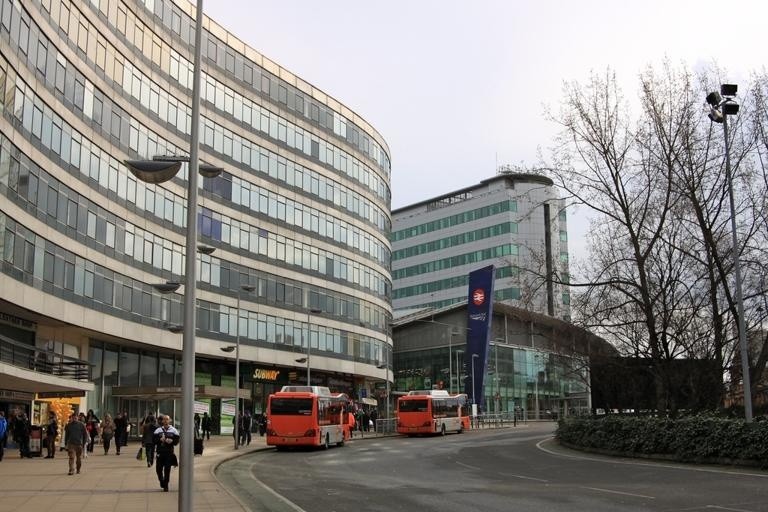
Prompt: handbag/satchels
<box><xmin>171</xmin><ymin>453</ymin><xmax>179</xmax><ymax>467</ymax></box>
<box><xmin>136</xmin><ymin>447</ymin><xmax>147</xmax><ymax>461</ymax></box>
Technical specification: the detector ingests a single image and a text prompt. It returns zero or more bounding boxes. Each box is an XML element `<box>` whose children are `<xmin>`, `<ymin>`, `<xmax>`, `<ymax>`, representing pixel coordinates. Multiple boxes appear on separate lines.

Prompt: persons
<box><xmin>152</xmin><ymin>415</ymin><xmax>180</xmax><ymax>492</ymax></box>
<box><xmin>64</xmin><ymin>413</ymin><xmax>88</xmax><ymax>474</ymax></box>
<box><xmin>349</xmin><ymin>408</ymin><xmax>376</xmax><ymax>438</ymax></box>
<box><xmin>1</xmin><ymin>405</ymin><xmax>266</xmax><ymax>467</ymax></box>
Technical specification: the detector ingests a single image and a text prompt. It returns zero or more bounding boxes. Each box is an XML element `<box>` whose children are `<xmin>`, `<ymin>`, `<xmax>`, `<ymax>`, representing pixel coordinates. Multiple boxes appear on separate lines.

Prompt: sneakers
<box><xmin>68</xmin><ymin>470</ymin><xmax>74</xmax><ymax>475</ymax></box>
<box><xmin>148</xmin><ymin>461</ymin><xmax>152</xmax><ymax>468</ymax></box>
<box><xmin>164</xmin><ymin>483</ymin><xmax>169</xmax><ymax>493</ymax></box>
<box><xmin>160</xmin><ymin>479</ymin><xmax>165</xmax><ymax>488</ymax></box>
<box><xmin>44</xmin><ymin>455</ymin><xmax>55</xmax><ymax>459</ymax></box>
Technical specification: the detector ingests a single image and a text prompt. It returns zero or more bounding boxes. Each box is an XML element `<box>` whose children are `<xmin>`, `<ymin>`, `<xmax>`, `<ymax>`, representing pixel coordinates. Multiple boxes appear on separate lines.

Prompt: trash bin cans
<box><xmin>194</xmin><ymin>438</ymin><xmax>203</xmax><ymax>456</ymax></box>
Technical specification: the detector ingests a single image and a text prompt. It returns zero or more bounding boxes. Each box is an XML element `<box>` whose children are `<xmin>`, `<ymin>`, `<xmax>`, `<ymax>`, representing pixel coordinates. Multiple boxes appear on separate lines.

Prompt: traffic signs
<box><xmin>439</xmin><ymin>381</ymin><xmax>443</xmax><ymax>388</ymax></box>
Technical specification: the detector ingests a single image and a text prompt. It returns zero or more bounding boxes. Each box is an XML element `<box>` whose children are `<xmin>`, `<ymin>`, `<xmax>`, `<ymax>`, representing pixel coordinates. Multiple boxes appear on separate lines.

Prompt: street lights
<box><xmin>704</xmin><ymin>81</ymin><xmax>754</xmax><ymax>420</ymax></box>
<box><xmin>456</xmin><ymin>348</ymin><xmax>464</xmax><ymax>396</ymax></box>
<box><xmin>120</xmin><ymin>152</ymin><xmax>224</xmax><ymax>511</ymax></box>
<box><xmin>489</xmin><ymin>337</ymin><xmax>504</xmax><ymax>427</ymax></box>
<box><xmin>219</xmin><ymin>284</ymin><xmax>254</xmax><ymax>450</ymax></box>
<box><xmin>449</xmin><ymin>332</ymin><xmax>461</xmax><ymax>397</ymax></box>
<box><xmin>471</xmin><ymin>353</ymin><xmax>480</xmax><ymax>405</ymax></box>
<box><xmin>376</xmin><ymin>322</ymin><xmax>397</xmax><ymax>433</ymax></box>
<box><xmin>533</xmin><ymin>353</ymin><xmax>540</xmax><ymax>419</ymax></box>
<box><xmin>294</xmin><ymin>306</ymin><xmax>323</xmax><ymax>388</ymax></box>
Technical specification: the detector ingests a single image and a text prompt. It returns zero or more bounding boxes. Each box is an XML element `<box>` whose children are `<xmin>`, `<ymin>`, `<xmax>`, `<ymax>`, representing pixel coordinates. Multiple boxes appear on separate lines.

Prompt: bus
<box><xmin>265</xmin><ymin>385</ymin><xmax>357</xmax><ymax>450</ymax></box>
<box><xmin>397</xmin><ymin>389</ymin><xmax>471</xmax><ymax>436</ymax></box>
